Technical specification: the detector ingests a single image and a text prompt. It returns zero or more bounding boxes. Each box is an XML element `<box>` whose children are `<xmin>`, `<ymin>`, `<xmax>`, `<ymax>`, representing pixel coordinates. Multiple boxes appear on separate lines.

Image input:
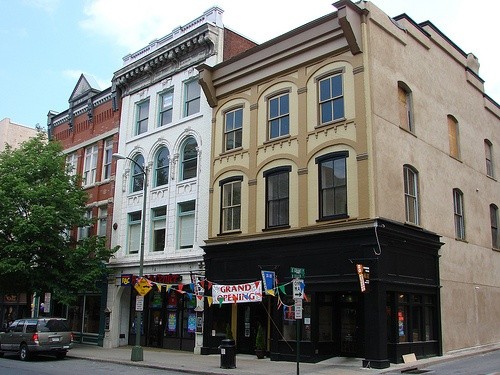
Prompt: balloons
<box><xmin>40</xmin><ymin>303</ymin><xmax>45</xmax><ymax>311</ymax></box>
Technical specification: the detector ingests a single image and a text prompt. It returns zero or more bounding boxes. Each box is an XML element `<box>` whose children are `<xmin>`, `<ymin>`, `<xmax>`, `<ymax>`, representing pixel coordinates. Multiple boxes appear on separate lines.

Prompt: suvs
<box><xmin>0</xmin><ymin>317</ymin><xmax>74</xmax><ymax>361</ymax></box>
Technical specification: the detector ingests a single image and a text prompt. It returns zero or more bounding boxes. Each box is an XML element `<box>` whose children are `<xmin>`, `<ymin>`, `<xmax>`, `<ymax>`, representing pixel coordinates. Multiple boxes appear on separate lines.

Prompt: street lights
<box><xmin>112</xmin><ymin>153</ymin><xmax>149</xmax><ymax>361</ymax></box>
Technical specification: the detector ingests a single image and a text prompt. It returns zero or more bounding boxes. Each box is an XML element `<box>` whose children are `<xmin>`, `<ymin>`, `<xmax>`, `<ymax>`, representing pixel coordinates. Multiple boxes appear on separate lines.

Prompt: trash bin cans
<box><xmin>220</xmin><ymin>339</ymin><xmax>236</xmax><ymax>369</ymax></box>
